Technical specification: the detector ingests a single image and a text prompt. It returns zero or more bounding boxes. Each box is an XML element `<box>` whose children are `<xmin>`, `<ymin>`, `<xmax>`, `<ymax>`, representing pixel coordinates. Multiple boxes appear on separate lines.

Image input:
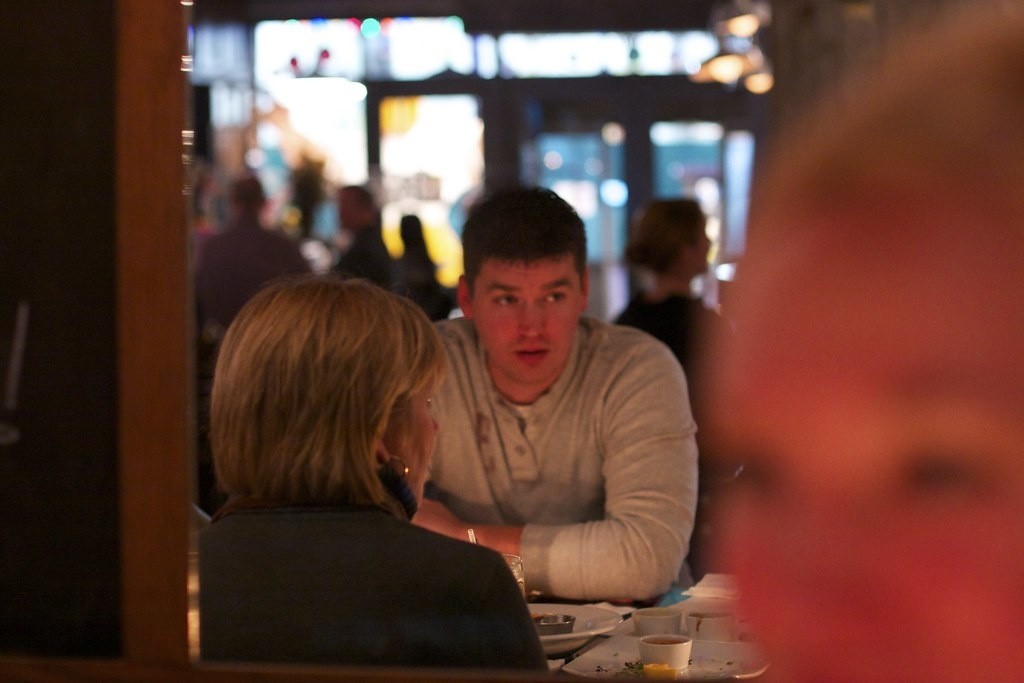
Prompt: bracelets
<box><xmin>467</xmin><ymin>527</ymin><xmax>476</xmax><ymax>544</ymax></box>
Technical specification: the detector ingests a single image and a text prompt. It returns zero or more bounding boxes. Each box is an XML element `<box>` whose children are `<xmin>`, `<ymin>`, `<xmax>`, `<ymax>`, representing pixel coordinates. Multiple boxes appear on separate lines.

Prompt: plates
<box><xmin>562</xmin><ymin>635</ymin><xmax>772</xmax><ymax>681</ymax></box>
<box><xmin>522</xmin><ymin>603</ymin><xmax>623</xmax><ymax>656</ymax></box>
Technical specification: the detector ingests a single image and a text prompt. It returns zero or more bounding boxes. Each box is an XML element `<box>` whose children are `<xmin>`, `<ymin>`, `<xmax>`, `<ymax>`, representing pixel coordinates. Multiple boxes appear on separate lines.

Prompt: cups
<box><xmin>496</xmin><ymin>553</ymin><xmax>525</xmax><ymax>602</ymax></box>
<box><xmin>632</xmin><ymin>609</ymin><xmax>682</xmax><ymax>638</ymax></box>
<box><xmin>637</xmin><ymin>635</ymin><xmax>692</xmax><ymax>672</ymax></box>
<box><xmin>685</xmin><ymin>611</ymin><xmax>736</xmax><ymax>642</ymax></box>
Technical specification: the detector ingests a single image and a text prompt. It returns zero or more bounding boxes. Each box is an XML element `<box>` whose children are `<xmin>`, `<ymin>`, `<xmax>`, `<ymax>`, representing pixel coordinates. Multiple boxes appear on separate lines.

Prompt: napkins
<box><xmin>682</xmin><ymin>573</ymin><xmax>743</xmax><ymax>602</ymax></box>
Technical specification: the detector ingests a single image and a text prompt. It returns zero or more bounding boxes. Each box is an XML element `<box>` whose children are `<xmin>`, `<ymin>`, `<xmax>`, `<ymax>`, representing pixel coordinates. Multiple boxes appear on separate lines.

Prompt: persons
<box><xmin>608</xmin><ymin>197</ymin><xmax>730</xmax><ymax>371</ymax></box>
<box><xmin>197</xmin><ymin>278</ymin><xmax>548</xmax><ymax>672</ymax></box>
<box><xmin>408</xmin><ymin>186</ymin><xmax>698</xmax><ymax>599</ymax></box>
<box><xmin>332</xmin><ymin>187</ymin><xmax>391</xmax><ymax>284</ymax></box>
<box><xmin>695</xmin><ymin>0</ymin><xmax>1023</xmax><ymax>683</ymax></box>
<box><xmin>199</xmin><ymin>176</ymin><xmax>306</xmax><ymax>354</ymax></box>
